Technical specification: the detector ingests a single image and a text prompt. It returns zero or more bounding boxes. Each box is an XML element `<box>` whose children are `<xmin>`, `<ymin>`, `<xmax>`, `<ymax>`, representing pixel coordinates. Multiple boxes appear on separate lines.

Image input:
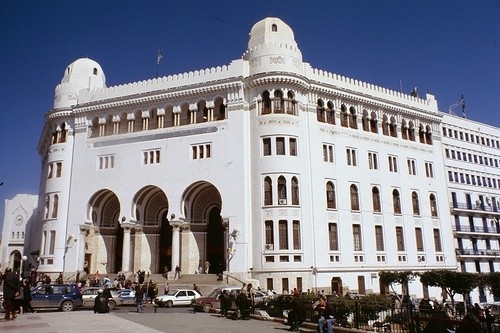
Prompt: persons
<box><xmin>386</xmin><ymin>292</ymin><xmax>415</xmax><ymax>309</ymax></box>
<box><xmin>0</xmin><ymin>266</ymin><xmax>63</xmax><ymax>320</ymax></box>
<box><xmin>164</xmin><ymin>282</ymin><xmax>169</xmax><ymax>294</ymax></box>
<box><xmin>318</xmin><ymin>296</ymin><xmax>326</xmax><ymax>316</ymax></box>
<box><xmin>283</xmin><ymin>287</ymin><xmax>325</xmax><ymax>296</ymax></box>
<box><xmin>174</xmin><ymin>265</ymin><xmax>181</xmax><ymax>279</ymax></box>
<box><xmin>134</xmin><ymin>269</ymin><xmax>157</xmax><ymax>312</ymax></box>
<box><xmin>164</xmin><ymin>265</ymin><xmax>168</xmax><ymax>276</ymax></box>
<box><xmin>76</xmin><ymin>270</ymin><xmax>132</xmax><ymax>313</ymax></box>
<box><xmin>420</xmin><ymin>298</ymin><xmax>447</xmax><ymax>310</ymax></box>
<box><xmin>218</xmin><ymin>283</ymin><xmax>254</xmax><ymax>312</ymax></box>
<box><xmin>194</xmin><ymin>284</ymin><xmax>200</xmax><ymax>293</ymax></box>
<box><xmin>199</xmin><ymin>260</ymin><xmax>211</xmax><ymax>273</ymax></box>
<box><xmin>473</xmin><ymin>302</ymin><xmax>495</xmax><ymax>323</ymax></box>
<box><xmin>258</xmin><ymin>287</ymin><xmax>276</xmax><ymax>295</ymax></box>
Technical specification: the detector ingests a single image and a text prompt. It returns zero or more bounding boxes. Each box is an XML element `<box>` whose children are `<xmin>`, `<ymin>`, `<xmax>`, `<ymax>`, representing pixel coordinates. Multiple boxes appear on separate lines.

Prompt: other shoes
<box><xmin>10</xmin><ymin>315</ymin><xmax>17</xmax><ymax>320</ymax></box>
<box><xmin>3</xmin><ymin>318</ymin><xmax>10</xmax><ymax>321</ymax></box>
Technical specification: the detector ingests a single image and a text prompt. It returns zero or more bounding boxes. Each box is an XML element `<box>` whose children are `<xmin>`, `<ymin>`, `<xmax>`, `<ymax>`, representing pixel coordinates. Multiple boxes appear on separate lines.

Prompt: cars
<box><xmin>3</xmin><ymin>284</ymin><xmax>83</xmax><ymax>312</ymax></box>
<box><xmin>154</xmin><ymin>289</ymin><xmax>202</xmax><ymax>308</ymax></box>
<box><xmin>418</xmin><ymin>299</ymin><xmax>500</xmax><ymax>322</ymax></box>
<box><xmin>76</xmin><ymin>286</ymin><xmax>124</xmax><ymax>310</ymax></box>
<box><xmin>193</xmin><ymin>288</ymin><xmax>272</xmax><ymax>313</ymax></box>
<box><xmin>115</xmin><ymin>289</ymin><xmax>148</xmax><ymax>307</ymax></box>
<box><xmin>393</xmin><ymin>294</ymin><xmax>416</xmax><ymax>311</ymax></box>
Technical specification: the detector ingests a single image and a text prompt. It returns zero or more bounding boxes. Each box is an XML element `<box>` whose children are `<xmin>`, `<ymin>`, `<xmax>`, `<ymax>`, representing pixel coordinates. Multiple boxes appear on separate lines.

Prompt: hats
<box><xmin>99</xmin><ymin>289</ymin><xmax>104</xmax><ymax>293</ymax></box>
